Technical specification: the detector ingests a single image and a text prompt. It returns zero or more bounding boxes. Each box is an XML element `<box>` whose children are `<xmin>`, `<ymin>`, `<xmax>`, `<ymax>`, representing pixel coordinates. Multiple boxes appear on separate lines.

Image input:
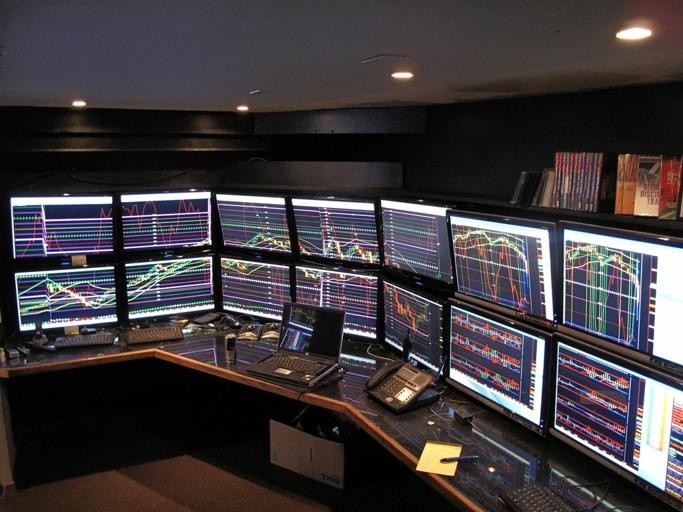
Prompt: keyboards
<box><xmin>498</xmin><ymin>479</ymin><xmax>575</xmax><ymax>512</ymax></box>
<box><xmin>126</xmin><ymin>325</ymin><xmax>184</xmax><ymax>345</ymax></box>
<box><xmin>54</xmin><ymin>332</ymin><xmax>115</xmax><ymax>348</ymax></box>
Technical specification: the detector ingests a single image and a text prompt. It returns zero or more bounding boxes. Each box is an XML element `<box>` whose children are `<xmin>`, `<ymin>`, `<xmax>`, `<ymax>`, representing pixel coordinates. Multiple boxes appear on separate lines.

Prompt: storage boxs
<box><xmin>269</xmin><ymin>418</ymin><xmax>371</xmax><ymax>490</ymax></box>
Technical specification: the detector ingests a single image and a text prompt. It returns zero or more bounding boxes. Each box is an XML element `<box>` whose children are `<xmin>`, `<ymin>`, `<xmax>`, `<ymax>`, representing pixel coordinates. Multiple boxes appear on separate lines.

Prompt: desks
<box><xmin>0</xmin><ymin>321</ymin><xmax>683</xmax><ymax>511</ymax></box>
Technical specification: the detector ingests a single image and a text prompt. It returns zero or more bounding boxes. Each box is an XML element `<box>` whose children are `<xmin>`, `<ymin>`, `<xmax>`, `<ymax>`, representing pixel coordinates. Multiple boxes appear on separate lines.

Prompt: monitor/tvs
<box><xmin>556</xmin><ymin>218</ymin><xmax>683</xmax><ymax>377</ymax></box>
<box><xmin>379</xmin><ymin>195</ymin><xmax>452</xmax><ymax>292</ymax></box>
<box><xmin>291</xmin><ymin>194</ymin><xmax>379</xmax><ymax>271</ymax></box>
<box><xmin>7</xmin><ymin>190</ymin><xmax>117</xmax><ymax>262</ymax></box>
<box><xmin>214</xmin><ymin>194</ymin><xmax>291</xmax><ymax>252</ymax></box>
<box><xmin>443</xmin><ymin>298</ymin><xmax>555</xmax><ymax>438</ymax></box>
<box><xmin>121</xmin><ymin>251</ymin><xmax>217</xmax><ymax>323</ymax></box>
<box><xmin>491</xmin><ymin>379</ymin><xmax>492</xmax><ymax>383</ymax></box>
<box><xmin>545</xmin><ymin>331</ymin><xmax>683</xmax><ymax>512</ymax></box>
<box><xmin>11</xmin><ymin>259</ymin><xmax>122</xmax><ymax>336</ymax></box>
<box><xmin>445</xmin><ymin>209</ymin><xmax>556</xmax><ymax>332</ymax></box>
<box><xmin>118</xmin><ymin>185</ymin><xmax>213</xmax><ymax>255</ymax></box>
<box><xmin>217</xmin><ymin>252</ymin><xmax>292</xmax><ymax>324</ymax></box>
<box><xmin>292</xmin><ymin>263</ymin><xmax>380</xmax><ymax>344</ymax></box>
<box><xmin>379</xmin><ymin>275</ymin><xmax>452</xmax><ymax>376</ymax></box>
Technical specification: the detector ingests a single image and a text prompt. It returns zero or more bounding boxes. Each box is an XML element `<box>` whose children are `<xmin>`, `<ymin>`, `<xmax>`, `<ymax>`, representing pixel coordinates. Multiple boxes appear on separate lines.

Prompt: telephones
<box><xmin>362</xmin><ymin>359</ymin><xmax>440</xmax><ymax>417</ymax></box>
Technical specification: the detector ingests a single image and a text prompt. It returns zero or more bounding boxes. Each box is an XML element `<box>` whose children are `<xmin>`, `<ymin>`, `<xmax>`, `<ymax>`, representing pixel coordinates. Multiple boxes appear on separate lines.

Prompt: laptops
<box><xmin>245</xmin><ymin>301</ymin><xmax>346</xmax><ymax>389</ymax></box>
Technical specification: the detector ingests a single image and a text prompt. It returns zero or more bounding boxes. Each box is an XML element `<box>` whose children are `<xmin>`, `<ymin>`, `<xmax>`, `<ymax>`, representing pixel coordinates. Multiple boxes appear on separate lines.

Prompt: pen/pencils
<box><xmin>441</xmin><ymin>455</ymin><xmax>480</xmax><ymax>464</ymax></box>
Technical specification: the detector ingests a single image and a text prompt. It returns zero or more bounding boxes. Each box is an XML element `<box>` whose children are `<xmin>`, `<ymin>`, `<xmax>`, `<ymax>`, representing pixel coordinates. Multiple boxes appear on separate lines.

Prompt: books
<box><xmin>614</xmin><ymin>152</ymin><xmax>683</xmax><ymax>221</ymax></box>
<box><xmin>510</xmin><ymin>151</ymin><xmax>610</xmax><ymax>213</ymax></box>
<box><xmin>414</xmin><ymin>440</ymin><xmax>463</xmax><ymax>477</ymax></box>
